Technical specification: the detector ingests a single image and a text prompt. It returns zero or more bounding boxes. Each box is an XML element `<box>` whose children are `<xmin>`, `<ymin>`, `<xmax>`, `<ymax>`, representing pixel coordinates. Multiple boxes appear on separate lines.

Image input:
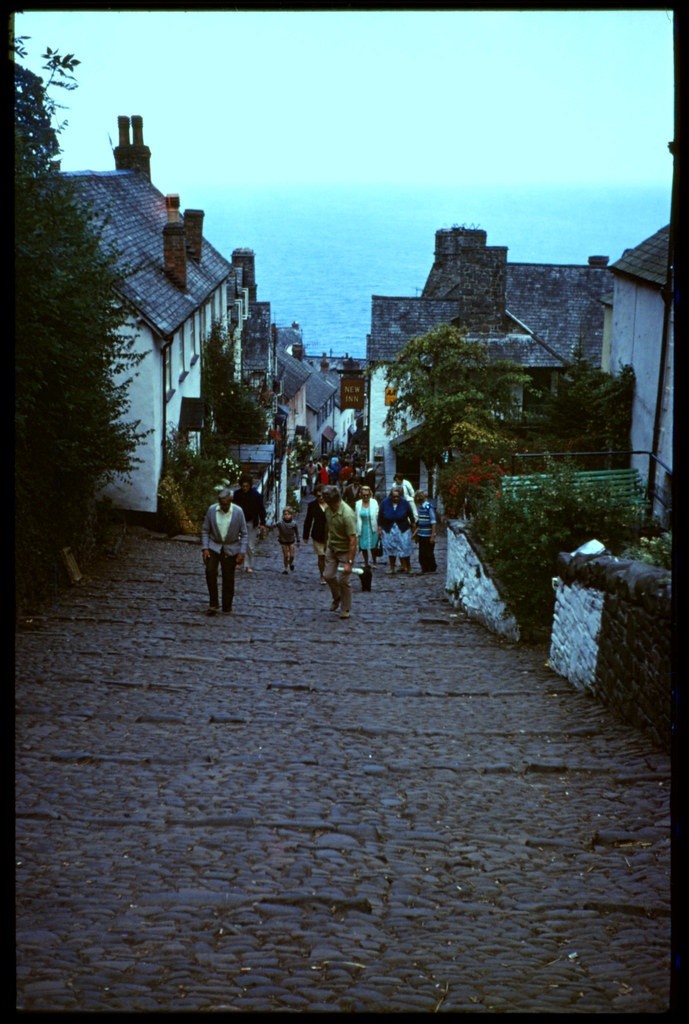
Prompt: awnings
<box><xmin>321</xmin><ymin>425</ymin><xmax>337</xmax><ymax>443</ymax></box>
<box><xmin>348</xmin><ymin>426</ymin><xmax>355</xmax><ymax>435</ymax></box>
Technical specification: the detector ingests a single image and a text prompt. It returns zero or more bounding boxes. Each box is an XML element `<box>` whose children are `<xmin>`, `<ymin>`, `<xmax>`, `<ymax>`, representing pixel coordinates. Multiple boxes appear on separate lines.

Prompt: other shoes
<box><xmin>330</xmin><ymin>597</ymin><xmax>341</xmax><ymax>611</ymax></box>
<box><xmin>223</xmin><ymin>610</ymin><xmax>231</xmax><ymax>614</ymax></box>
<box><xmin>372</xmin><ymin>565</ymin><xmax>376</xmax><ymax>568</ymax></box>
<box><xmin>235</xmin><ymin>564</ymin><xmax>240</xmax><ymax>572</ymax></box>
<box><xmin>407</xmin><ymin>569</ymin><xmax>414</xmax><ymax>575</ymax></box>
<box><xmin>340</xmin><ymin>609</ymin><xmax>350</xmax><ymax>618</ymax></box>
<box><xmin>397</xmin><ymin>566</ymin><xmax>404</xmax><ymax>572</ymax></box>
<box><xmin>289</xmin><ymin>561</ymin><xmax>295</xmax><ymax>571</ymax></box>
<box><xmin>207</xmin><ymin>605</ymin><xmax>219</xmax><ymax>612</ymax></box>
<box><xmin>320</xmin><ymin>577</ymin><xmax>327</xmax><ymax>584</ymax></box>
<box><xmin>385</xmin><ymin>569</ymin><xmax>395</xmax><ymax>575</ymax></box>
<box><xmin>417</xmin><ymin>570</ymin><xmax>427</xmax><ymax>575</ymax></box>
<box><xmin>244</xmin><ymin>567</ymin><xmax>253</xmax><ymax>572</ymax></box>
<box><xmin>282</xmin><ymin>568</ymin><xmax>288</xmax><ymax>574</ymax></box>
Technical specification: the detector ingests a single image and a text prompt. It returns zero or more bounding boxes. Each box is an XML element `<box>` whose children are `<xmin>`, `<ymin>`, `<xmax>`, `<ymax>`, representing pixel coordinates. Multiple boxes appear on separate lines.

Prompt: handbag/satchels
<box><xmin>374</xmin><ymin>538</ymin><xmax>382</xmax><ymax>557</ymax></box>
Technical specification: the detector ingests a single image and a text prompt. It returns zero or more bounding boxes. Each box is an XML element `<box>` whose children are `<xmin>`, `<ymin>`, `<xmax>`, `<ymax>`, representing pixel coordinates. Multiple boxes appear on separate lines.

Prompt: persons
<box><xmin>232</xmin><ymin>447</ymin><xmax>438</xmax><ymax>592</ymax></box>
<box><xmin>321</xmin><ymin>484</ymin><xmax>358</xmax><ymax>617</ymax></box>
<box><xmin>201</xmin><ymin>489</ymin><xmax>249</xmax><ymax>614</ymax></box>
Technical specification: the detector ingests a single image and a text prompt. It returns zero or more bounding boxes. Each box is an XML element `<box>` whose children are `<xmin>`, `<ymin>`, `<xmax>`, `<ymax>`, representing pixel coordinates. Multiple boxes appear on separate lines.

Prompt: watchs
<box><xmin>347</xmin><ymin>560</ymin><xmax>353</xmax><ymax>564</ymax></box>
